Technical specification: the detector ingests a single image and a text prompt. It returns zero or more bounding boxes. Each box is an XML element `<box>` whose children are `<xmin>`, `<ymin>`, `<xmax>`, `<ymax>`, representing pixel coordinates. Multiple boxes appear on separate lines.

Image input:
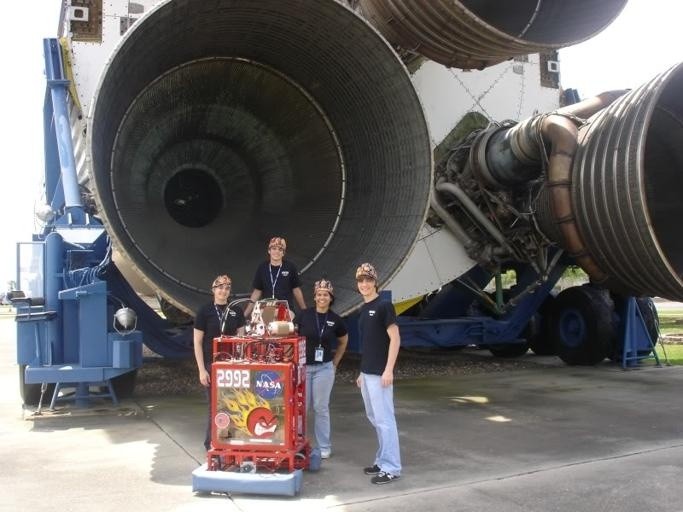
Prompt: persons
<box><xmin>192</xmin><ymin>272</ymin><xmax>248</xmax><ymax>454</ymax></box>
<box><xmin>243</xmin><ymin>235</ymin><xmax>308</xmax><ymax>324</ymax></box>
<box><xmin>292</xmin><ymin>275</ymin><xmax>350</xmax><ymax>462</ymax></box>
<box><xmin>354</xmin><ymin>262</ymin><xmax>403</xmax><ymax>485</ymax></box>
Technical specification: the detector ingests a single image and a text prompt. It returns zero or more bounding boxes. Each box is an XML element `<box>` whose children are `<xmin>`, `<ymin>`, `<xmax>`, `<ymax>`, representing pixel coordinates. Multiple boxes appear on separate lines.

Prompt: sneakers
<box><xmin>363</xmin><ymin>465</ymin><xmax>381</xmax><ymax>475</ymax></box>
<box><xmin>320</xmin><ymin>448</ymin><xmax>333</xmax><ymax>461</ymax></box>
<box><xmin>370</xmin><ymin>471</ymin><xmax>401</xmax><ymax>485</ymax></box>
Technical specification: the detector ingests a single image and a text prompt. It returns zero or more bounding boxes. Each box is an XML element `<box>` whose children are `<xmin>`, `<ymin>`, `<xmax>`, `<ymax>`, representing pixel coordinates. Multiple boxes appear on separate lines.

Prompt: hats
<box><xmin>267</xmin><ymin>236</ymin><xmax>288</xmax><ymax>252</ymax></box>
<box><xmin>355</xmin><ymin>262</ymin><xmax>378</xmax><ymax>281</ymax></box>
<box><xmin>312</xmin><ymin>278</ymin><xmax>334</xmax><ymax>295</ymax></box>
<box><xmin>212</xmin><ymin>274</ymin><xmax>233</xmax><ymax>288</ymax></box>
